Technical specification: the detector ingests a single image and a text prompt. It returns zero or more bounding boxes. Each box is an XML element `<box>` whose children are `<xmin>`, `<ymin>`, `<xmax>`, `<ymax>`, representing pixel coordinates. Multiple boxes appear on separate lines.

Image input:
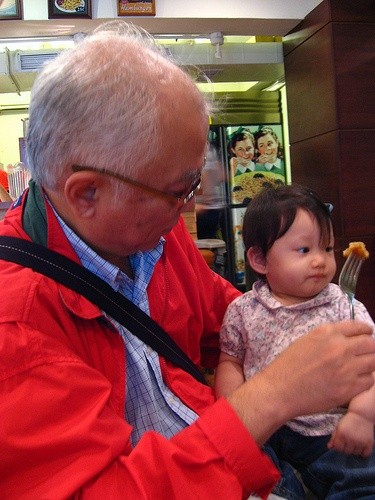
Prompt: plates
<box><xmin>55</xmin><ymin>0</ymin><xmax>86</xmax><ymax>12</ymax></box>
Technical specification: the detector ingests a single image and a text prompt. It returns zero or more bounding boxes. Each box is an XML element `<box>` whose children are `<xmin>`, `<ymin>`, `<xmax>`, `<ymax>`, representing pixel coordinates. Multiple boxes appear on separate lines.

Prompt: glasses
<box><xmin>71</xmin><ymin>154</ymin><xmax>207</xmax><ymax>209</ymax></box>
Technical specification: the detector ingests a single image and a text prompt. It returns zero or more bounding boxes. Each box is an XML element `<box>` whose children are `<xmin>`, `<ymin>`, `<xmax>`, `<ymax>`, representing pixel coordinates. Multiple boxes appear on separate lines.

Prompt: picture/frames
<box><xmin>116</xmin><ymin>0</ymin><xmax>156</xmax><ymax>17</ymax></box>
<box><xmin>48</xmin><ymin>0</ymin><xmax>92</xmax><ymax>19</ymax></box>
<box><xmin>0</xmin><ymin>0</ymin><xmax>23</xmax><ymax>21</ymax></box>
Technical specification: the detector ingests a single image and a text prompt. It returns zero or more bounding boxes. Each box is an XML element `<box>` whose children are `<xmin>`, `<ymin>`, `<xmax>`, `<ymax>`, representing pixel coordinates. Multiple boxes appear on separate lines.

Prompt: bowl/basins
<box><xmin>232</xmin><ymin>171</ymin><xmax>285</xmax><ymax>187</ymax></box>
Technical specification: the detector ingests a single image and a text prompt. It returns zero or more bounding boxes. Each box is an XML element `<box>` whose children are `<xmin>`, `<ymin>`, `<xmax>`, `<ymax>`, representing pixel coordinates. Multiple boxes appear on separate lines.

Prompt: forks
<box><xmin>338</xmin><ymin>250</ymin><xmax>365</xmax><ymax>320</ymax></box>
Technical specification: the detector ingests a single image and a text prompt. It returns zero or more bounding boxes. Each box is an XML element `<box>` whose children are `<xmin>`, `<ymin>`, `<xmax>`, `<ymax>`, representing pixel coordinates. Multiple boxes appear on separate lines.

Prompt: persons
<box><xmin>213</xmin><ymin>186</ymin><xmax>375</xmax><ymax>500</ymax></box>
<box><xmin>253</xmin><ymin>127</ymin><xmax>285</xmax><ymax>176</ymax></box>
<box><xmin>0</xmin><ymin>19</ymin><xmax>375</xmax><ymax>499</ymax></box>
<box><xmin>196</xmin><ymin>139</ymin><xmax>228</xmax><ymax>279</ymax></box>
<box><xmin>228</xmin><ymin>131</ymin><xmax>272</xmax><ymax>176</ymax></box>
